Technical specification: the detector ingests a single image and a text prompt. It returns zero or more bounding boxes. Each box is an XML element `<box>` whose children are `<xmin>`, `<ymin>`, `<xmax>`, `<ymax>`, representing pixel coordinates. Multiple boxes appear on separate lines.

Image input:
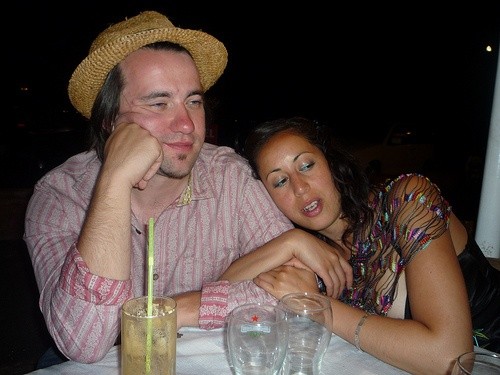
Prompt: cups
<box><xmin>121</xmin><ymin>296</ymin><xmax>177</xmax><ymax>375</ymax></box>
<box><xmin>279</xmin><ymin>293</ymin><xmax>332</xmax><ymax>375</ymax></box>
<box><xmin>451</xmin><ymin>352</ymin><xmax>500</xmax><ymax>375</ymax></box>
<box><xmin>227</xmin><ymin>303</ymin><xmax>288</xmax><ymax>375</ymax></box>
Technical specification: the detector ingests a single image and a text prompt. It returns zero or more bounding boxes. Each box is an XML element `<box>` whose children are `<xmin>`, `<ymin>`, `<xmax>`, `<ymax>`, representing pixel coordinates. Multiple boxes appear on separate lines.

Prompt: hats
<box><xmin>68</xmin><ymin>11</ymin><xmax>227</xmax><ymax>119</ymax></box>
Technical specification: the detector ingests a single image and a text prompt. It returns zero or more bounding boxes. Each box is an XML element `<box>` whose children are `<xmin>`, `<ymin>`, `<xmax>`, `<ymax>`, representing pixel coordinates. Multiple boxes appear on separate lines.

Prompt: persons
<box><xmin>215</xmin><ymin>115</ymin><xmax>500</xmax><ymax>375</ymax></box>
<box><xmin>23</xmin><ymin>11</ymin><xmax>318</xmax><ymax>364</ymax></box>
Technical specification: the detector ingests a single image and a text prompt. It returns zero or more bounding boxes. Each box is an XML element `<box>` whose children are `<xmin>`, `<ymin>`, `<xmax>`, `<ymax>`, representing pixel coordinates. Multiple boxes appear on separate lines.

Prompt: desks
<box><xmin>24</xmin><ymin>315</ymin><xmax>500</xmax><ymax>375</ymax></box>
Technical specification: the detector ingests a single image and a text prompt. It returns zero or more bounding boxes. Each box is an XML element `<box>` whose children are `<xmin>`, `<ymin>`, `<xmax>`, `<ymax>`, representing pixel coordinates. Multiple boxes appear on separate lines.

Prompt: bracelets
<box><xmin>354</xmin><ymin>312</ymin><xmax>370</xmax><ymax>353</ymax></box>
